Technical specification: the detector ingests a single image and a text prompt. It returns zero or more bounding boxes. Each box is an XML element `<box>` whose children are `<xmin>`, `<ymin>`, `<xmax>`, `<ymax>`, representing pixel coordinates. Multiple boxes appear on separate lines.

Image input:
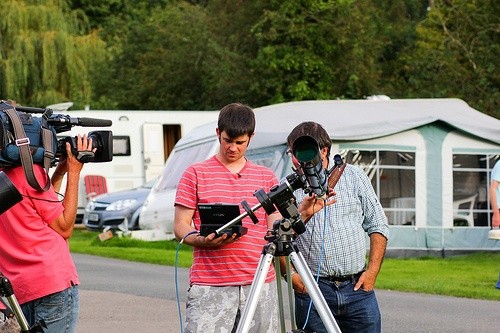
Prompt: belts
<box><xmin>313</xmin><ymin>270</ymin><xmax>365</xmax><ymax>282</ymax></box>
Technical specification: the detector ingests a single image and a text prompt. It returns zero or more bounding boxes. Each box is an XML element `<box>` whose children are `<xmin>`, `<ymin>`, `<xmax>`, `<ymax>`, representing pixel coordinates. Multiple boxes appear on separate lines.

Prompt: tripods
<box><xmin>234</xmin><ymin>219</ymin><xmax>344</xmax><ymax>333</ymax></box>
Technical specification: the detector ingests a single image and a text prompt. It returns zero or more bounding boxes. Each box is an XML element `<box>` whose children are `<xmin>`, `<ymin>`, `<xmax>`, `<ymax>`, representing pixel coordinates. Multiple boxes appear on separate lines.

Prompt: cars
<box><xmin>83</xmin><ymin>175</ymin><xmax>160</xmax><ymax>229</ymax></box>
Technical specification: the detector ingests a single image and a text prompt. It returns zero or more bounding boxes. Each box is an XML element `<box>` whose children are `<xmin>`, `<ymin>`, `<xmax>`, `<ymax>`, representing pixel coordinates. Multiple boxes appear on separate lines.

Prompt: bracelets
<box><xmin>55</xmin><ymin>170</ymin><xmax>65</xmax><ymax>175</ymax></box>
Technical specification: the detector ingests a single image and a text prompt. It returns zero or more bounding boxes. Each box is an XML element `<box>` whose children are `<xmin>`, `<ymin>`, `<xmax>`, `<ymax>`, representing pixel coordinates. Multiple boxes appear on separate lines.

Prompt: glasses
<box><xmin>286</xmin><ymin>148</ymin><xmax>294</xmax><ymax>158</ymax></box>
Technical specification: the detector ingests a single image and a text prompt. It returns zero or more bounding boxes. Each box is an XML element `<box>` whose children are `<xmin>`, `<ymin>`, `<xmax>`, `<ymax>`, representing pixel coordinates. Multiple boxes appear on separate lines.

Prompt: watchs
<box><xmin>284</xmin><ymin>269</ymin><xmax>297</xmax><ymax>282</ymax></box>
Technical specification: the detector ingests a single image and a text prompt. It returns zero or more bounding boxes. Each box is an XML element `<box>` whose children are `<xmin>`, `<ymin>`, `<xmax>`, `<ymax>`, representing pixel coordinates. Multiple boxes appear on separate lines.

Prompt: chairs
<box><xmin>454</xmin><ymin>193</ymin><xmax>477</xmax><ymax>227</ymax></box>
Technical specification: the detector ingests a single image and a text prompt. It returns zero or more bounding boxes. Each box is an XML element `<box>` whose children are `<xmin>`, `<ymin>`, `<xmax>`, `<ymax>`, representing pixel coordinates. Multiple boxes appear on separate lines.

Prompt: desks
<box><xmin>389</xmin><ymin>196</ymin><xmax>415</xmax><ymax>224</ymax></box>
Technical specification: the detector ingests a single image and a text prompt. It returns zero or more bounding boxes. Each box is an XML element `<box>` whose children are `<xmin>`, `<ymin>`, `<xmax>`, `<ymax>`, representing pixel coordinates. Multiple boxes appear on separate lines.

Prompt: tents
<box><xmin>138</xmin><ymin>95</ymin><xmax>500</xmax><ymax>260</ymax></box>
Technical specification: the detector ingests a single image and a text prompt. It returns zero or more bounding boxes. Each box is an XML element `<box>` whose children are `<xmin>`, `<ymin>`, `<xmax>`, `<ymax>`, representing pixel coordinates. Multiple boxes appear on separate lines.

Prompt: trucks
<box><xmin>29</xmin><ymin>110</ymin><xmax>221</xmax><ymax>223</ymax></box>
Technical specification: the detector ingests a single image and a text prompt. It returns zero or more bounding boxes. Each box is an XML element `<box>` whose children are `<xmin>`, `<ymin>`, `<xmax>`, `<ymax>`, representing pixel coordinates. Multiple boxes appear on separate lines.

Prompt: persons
<box><xmin>272</xmin><ymin>121</ymin><xmax>389</xmax><ymax>333</ymax></box>
<box><xmin>174</xmin><ymin>102</ymin><xmax>337</xmax><ymax>333</ymax></box>
<box><xmin>489</xmin><ymin>159</ymin><xmax>500</xmax><ymax>290</ymax></box>
<box><xmin>0</xmin><ymin>99</ymin><xmax>96</xmax><ymax>333</ymax></box>
